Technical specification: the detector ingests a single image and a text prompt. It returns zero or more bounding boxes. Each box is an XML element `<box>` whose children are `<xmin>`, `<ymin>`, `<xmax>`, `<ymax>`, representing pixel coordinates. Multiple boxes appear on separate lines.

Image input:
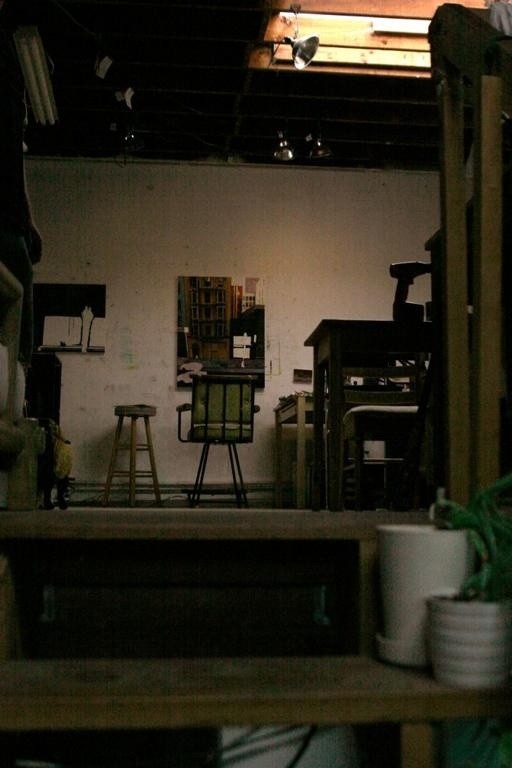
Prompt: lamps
<box><xmin>285</xmin><ymin>36</ymin><xmax>320</xmax><ymax>70</ymax></box>
<box><xmin>271</xmin><ymin>121</ymin><xmax>334</xmax><ymax>162</ymax></box>
<box><xmin>12</xmin><ymin>22</ymin><xmax>58</xmax><ymax>125</ymax></box>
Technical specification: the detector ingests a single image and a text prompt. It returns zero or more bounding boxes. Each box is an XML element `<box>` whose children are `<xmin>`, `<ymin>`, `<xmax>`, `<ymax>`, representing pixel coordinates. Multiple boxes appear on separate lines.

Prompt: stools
<box><xmin>102</xmin><ymin>405</ymin><xmax>161</xmax><ymax>508</ymax></box>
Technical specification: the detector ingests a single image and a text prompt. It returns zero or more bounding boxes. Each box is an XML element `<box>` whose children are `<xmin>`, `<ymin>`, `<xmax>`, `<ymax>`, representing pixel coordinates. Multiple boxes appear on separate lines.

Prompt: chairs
<box><xmin>176</xmin><ymin>373</ymin><xmax>260</xmax><ymax>509</ymax></box>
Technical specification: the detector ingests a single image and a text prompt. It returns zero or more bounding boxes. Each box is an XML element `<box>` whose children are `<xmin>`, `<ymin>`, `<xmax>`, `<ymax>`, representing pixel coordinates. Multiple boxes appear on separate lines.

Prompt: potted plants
<box><xmin>374</xmin><ymin>472</ymin><xmax>512</xmax><ymax>690</ymax></box>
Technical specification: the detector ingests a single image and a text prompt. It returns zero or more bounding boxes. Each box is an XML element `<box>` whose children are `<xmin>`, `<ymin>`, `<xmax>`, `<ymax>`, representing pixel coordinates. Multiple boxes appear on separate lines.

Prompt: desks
<box><xmin>272</xmin><ymin>319</ymin><xmax>434</xmax><ymax>511</ymax></box>
